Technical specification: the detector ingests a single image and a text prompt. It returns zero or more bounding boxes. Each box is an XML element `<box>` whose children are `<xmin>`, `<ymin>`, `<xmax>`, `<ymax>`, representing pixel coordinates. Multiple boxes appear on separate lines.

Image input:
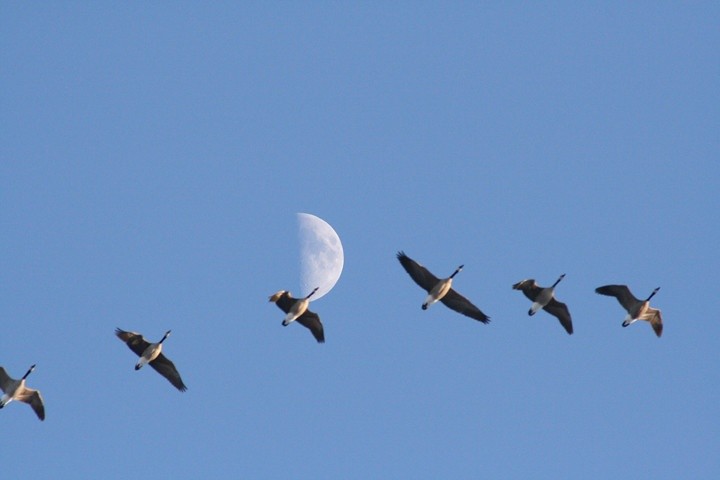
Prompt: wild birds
<box><xmin>511</xmin><ymin>273</ymin><xmax>574</xmax><ymax>335</ymax></box>
<box><xmin>267</xmin><ymin>286</ymin><xmax>326</xmax><ymax>344</ymax></box>
<box><xmin>594</xmin><ymin>284</ymin><xmax>664</xmax><ymax>338</ymax></box>
<box><xmin>0</xmin><ymin>363</ymin><xmax>46</xmax><ymax>421</ymax></box>
<box><xmin>114</xmin><ymin>326</ymin><xmax>188</xmax><ymax>393</ymax></box>
<box><xmin>395</xmin><ymin>249</ymin><xmax>491</xmax><ymax>325</ymax></box>
